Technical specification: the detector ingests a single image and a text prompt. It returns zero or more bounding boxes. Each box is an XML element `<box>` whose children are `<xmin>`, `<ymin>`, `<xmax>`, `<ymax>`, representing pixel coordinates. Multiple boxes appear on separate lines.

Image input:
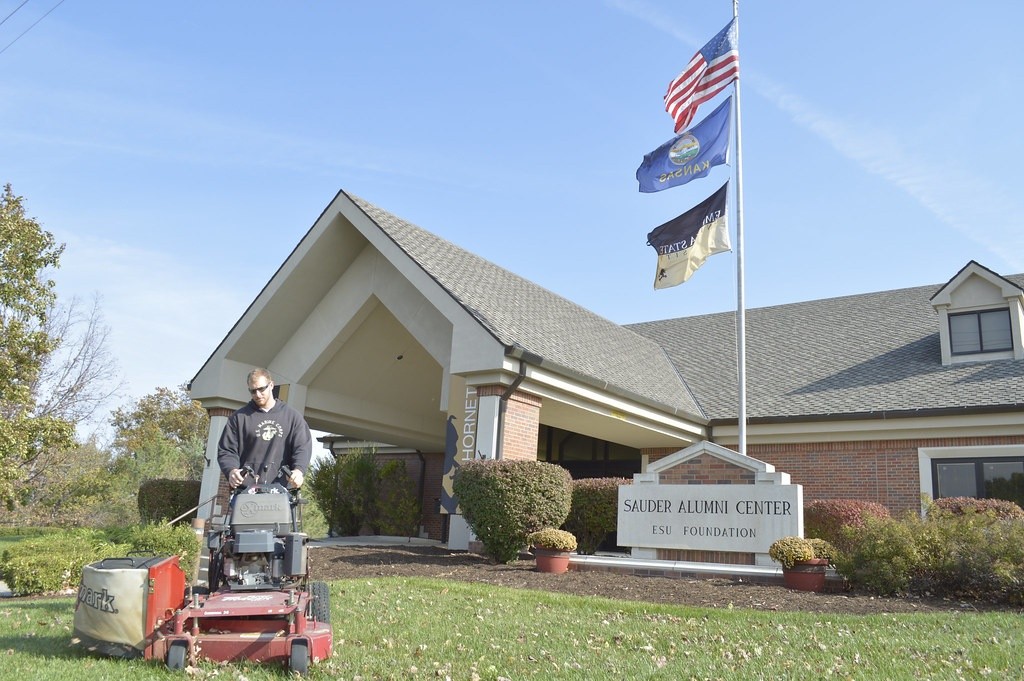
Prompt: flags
<box><xmin>636</xmin><ymin>96</ymin><xmax>733</xmax><ymax>193</ymax></box>
<box><xmin>646</xmin><ymin>180</ymin><xmax>732</xmax><ymax>291</ymax></box>
<box><xmin>663</xmin><ymin>16</ymin><xmax>739</xmax><ymax>133</ymax></box>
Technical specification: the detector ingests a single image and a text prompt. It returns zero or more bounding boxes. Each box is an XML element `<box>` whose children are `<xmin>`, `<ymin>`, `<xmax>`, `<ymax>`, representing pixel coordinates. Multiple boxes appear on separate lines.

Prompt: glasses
<box><xmin>249</xmin><ymin>382</ymin><xmax>271</xmax><ymax>394</ymax></box>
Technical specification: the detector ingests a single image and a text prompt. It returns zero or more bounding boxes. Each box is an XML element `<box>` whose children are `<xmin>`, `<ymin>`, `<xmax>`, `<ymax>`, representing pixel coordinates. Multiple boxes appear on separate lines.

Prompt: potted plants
<box><xmin>527</xmin><ymin>529</ymin><xmax>578</xmax><ymax>574</ymax></box>
<box><xmin>769</xmin><ymin>537</ymin><xmax>835</xmax><ymax>593</ymax></box>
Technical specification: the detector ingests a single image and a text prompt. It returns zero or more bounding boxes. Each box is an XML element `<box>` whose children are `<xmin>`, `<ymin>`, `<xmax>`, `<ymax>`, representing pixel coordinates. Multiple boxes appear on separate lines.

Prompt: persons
<box><xmin>217</xmin><ymin>368</ymin><xmax>312</xmax><ymax>490</ymax></box>
